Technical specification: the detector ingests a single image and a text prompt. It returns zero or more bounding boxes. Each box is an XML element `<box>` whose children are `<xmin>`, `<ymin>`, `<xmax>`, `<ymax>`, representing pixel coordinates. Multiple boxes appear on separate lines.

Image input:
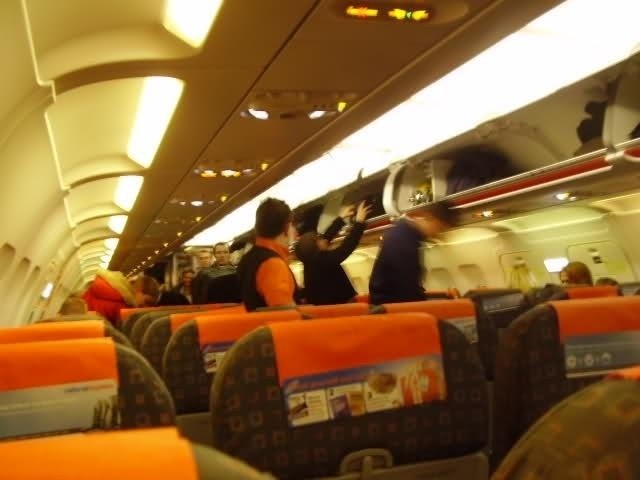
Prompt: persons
<box><xmin>196</xmin><ymin>242</ymin><xmax>236</xmax><ymax>302</ymax></box>
<box><xmin>559</xmin><ymin>261</ymin><xmax>592</xmax><ymax>288</ymax></box>
<box><xmin>366</xmin><ymin>204</ymin><xmax>459</xmax><ymax>304</ymax></box>
<box><xmin>61</xmin><ymin>295</ymin><xmax>87</xmax><ymax>314</ymax></box>
<box><xmin>83</xmin><ymin>268</ymin><xmax>158</xmax><ymax>326</ymax></box>
<box><xmin>154</xmin><ymin>288</ymin><xmax>190</xmax><ymax>307</ymax></box>
<box><xmin>295</xmin><ymin>201</ymin><xmax>372</xmax><ymax>304</ymax></box>
<box><xmin>173</xmin><ymin>271</ymin><xmax>195</xmax><ymax>302</ymax></box>
<box><xmin>192</xmin><ymin>250</ymin><xmax>215</xmax><ymax>301</ymax></box>
<box><xmin>235</xmin><ymin>199</ymin><xmax>300</xmax><ymax>312</ymax></box>
